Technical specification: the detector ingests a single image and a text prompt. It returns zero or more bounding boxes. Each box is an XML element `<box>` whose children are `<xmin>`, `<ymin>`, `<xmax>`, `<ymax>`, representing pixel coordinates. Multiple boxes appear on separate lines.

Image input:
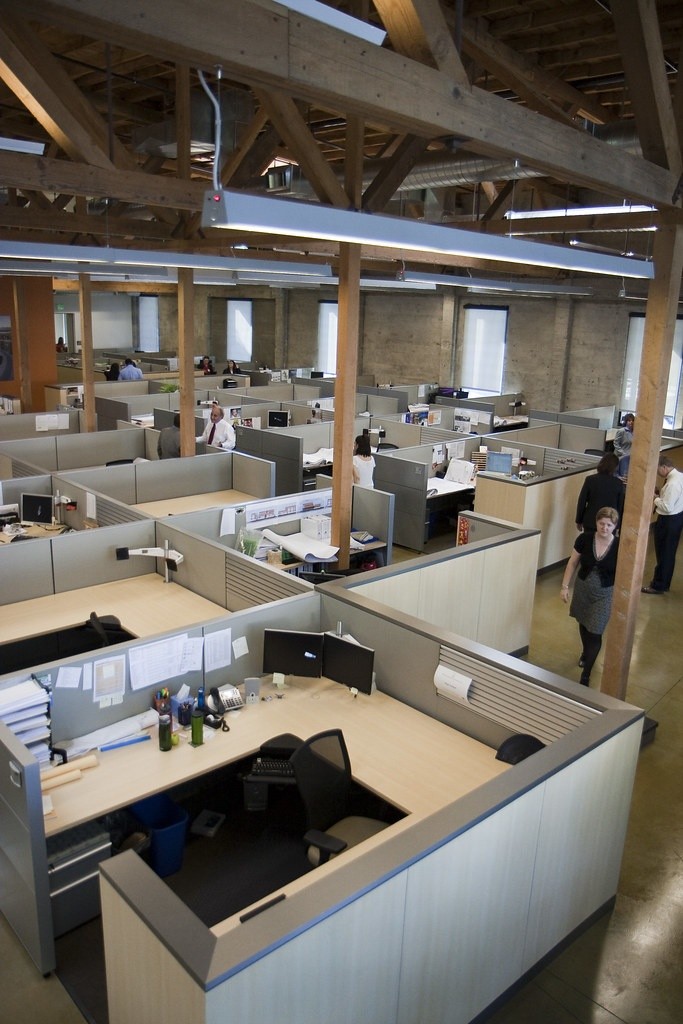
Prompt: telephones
<box><xmin>211</xmin><ymin>687</ymin><xmax>244</xmax><ymax>714</ymax></box>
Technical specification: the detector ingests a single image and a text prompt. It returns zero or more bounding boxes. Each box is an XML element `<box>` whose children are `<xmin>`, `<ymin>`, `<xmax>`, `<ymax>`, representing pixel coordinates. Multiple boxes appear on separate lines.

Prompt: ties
<box><xmin>208</xmin><ymin>423</ymin><xmax>215</xmax><ymax>444</ymax></box>
<box><xmin>653</xmin><ymin>479</ymin><xmax>667</xmax><ymax>514</ymax></box>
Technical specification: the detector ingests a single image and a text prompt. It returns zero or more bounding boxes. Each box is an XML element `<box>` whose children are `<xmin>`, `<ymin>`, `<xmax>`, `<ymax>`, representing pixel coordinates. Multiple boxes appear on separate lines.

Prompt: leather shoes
<box><xmin>664</xmin><ymin>587</ymin><xmax>670</xmax><ymax>591</ymax></box>
<box><xmin>641</xmin><ymin>586</ymin><xmax>664</xmax><ymax>594</ymax></box>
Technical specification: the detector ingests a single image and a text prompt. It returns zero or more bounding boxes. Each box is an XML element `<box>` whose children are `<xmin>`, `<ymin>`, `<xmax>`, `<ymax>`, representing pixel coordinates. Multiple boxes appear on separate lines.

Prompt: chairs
<box><xmin>290</xmin><ymin>729</ymin><xmax>402</xmax><ymax>867</ymax></box>
<box><xmin>90</xmin><ymin>611</ymin><xmax>129</xmax><ymax>648</ymax></box>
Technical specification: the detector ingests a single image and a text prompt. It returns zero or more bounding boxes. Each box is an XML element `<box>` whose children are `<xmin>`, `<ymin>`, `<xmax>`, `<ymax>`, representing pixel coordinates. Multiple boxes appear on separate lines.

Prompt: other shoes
<box><xmin>580</xmin><ymin>671</ymin><xmax>590</xmax><ymax>687</ymax></box>
<box><xmin>579</xmin><ymin>659</ymin><xmax>586</xmax><ymax>666</ymax></box>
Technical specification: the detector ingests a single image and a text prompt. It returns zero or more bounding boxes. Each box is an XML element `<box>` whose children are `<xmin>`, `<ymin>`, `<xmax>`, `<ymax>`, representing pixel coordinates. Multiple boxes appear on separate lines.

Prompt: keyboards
<box><xmin>251</xmin><ymin>757</ymin><xmax>296</xmax><ymax>776</ymax></box>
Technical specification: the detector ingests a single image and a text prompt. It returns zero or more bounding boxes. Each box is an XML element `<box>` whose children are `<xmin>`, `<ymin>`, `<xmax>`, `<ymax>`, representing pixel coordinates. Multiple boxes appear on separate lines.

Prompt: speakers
<box><xmin>244</xmin><ymin>677</ymin><xmax>261</xmax><ymax>703</ymax></box>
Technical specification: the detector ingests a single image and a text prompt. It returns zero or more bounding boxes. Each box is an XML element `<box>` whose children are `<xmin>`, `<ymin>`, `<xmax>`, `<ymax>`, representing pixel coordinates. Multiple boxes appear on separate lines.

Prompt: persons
<box><xmin>56</xmin><ymin>337</ymin><xmax>67</xmax><ymax>353</ymax></box>
<box><xmin>223</xmin><ymin>360</ymin><xmax>242</xmax><ymax>375</ymax></box>
<box><xmin>197</xmin><ymin>356</ymin><xmax>213</xmax><ymax>374</ymax></box>
<box><xmin>157</xmin><ymin>414</ymin><xmax>181</xmax><ymax>460</ymax></box>
<box><xmin>283</xmin><ymin>371</ymin><xmax>287</xmax><ymax>379</ymax></box>
<box><xmin>195</xmin><ymin>407</ymin><xmax>236</xmax><ymax>450</ymax></box>
<box><xmin>560</xmin><ymin>507</ymin><xmax>620</xmax><ymax>689</ymax></box>
<box><xmin>613</xmin><ymin>413</ymin><xmax>634</xmax><ymax>480</ymax></box>
<box><xmin>104</xmin><ymin>358</ymin><xmax>143</xmax><ymax>381</ymax></box>
<box><xmin>641</xmin><ymin>454</ymin><xmax>683</xmax><ymax>594</ymax></box>
<box><xmin>352</xmin><ymin>435</ymin><xmax>377</xmax><ymax>489</ymax></box>
<box><xmin>231</xmin><ymin>409</ymin><xmax>239</xmax><ymax>418</ymax></box>
<box><xmin>575</xmin><ymin>451</ymin><xmax>626</xmax><ymax>533</ymax></box>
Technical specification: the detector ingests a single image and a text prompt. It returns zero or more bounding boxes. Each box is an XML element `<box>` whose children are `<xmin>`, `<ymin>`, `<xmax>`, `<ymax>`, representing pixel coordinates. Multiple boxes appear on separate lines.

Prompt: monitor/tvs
<box><xmin>485</xmin><ymin>451</ymin><xmax>512</xmax><ymax>476</ymax></box>
<box><xmin>310</xmin><ymin>372</ymin><xmax>324</xmax><ymax>379</ymax></box>
<box><xmin>299</xmin><ymin>571</ymin><xmax>345</xmax><ymax>585</ymax></box>
<box><xmin>322</xmin><ymin>632</ymin><xmax>375</xmax><ymax>696</ymax></box>
<box><xmin>263</xmin><ymin>628</ymin><xmax>323</xmax><ymax>678</ymax></box>
<box><xmin>20</xmin><ymin>493</ymin><xmax>54</xmax><ymax>525</ymax></box>
<box><xmin>267</xmin><ymin>410</ymin><xmax>290</xmax><ymax>429</ymax></box>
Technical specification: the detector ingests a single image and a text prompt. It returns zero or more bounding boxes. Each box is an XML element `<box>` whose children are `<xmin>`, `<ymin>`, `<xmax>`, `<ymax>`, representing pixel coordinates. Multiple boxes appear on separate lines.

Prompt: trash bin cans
<box><xmin>98</xmin><ymin>808</ymin><xmax>152</xmax><ymax>873</ymax></box>
<box><xmin>135</xmin><ymin>793</ymin><xmax>189</xmax><ymax>876</ymax></box>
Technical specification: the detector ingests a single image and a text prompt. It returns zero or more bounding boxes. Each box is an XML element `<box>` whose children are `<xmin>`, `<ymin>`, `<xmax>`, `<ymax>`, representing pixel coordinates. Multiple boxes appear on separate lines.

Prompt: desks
<box><xmin>0</xmin><ymin>339</ymin><xmax>682</xmax><ymax>1023</ymax></box>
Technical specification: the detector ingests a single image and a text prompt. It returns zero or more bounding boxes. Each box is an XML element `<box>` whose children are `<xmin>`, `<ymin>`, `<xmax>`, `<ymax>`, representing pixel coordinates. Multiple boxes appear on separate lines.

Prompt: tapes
<box><xmin>208</xmin><ymin>714</ymin><xmax>220</xmax><ymax>722</ymax></box>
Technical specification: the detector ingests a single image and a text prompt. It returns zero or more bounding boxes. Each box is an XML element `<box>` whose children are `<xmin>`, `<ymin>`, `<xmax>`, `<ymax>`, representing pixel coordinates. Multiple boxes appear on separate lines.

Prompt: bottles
<box><xmin>191</xmin><ymin>710</ymin><xmax>204</xmax><ymax>744</ymax></box>
<box><xmin>158</xmin><ymin>716</ymin><xmax>171</xmax><ymax>751</ymax></box>
<box><xmin>159</xmin><ymin>703</ymin><xmax>173</xmax><ymax>735</ymax></box>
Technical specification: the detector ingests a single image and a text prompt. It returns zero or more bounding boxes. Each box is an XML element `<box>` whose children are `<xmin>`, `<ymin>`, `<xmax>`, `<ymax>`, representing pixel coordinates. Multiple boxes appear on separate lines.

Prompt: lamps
<box><xmin>0</xmin><ymin>70</ymin><xmax>657</xmax><ymax>289</ymax></box>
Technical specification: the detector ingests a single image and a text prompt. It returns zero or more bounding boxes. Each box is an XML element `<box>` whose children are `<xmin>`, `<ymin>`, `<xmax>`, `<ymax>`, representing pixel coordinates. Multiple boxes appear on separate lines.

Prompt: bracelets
<box><xmin>561</xmin><ymin>584</ymin><xmax>569</xmax><ymax>588</ymax></box>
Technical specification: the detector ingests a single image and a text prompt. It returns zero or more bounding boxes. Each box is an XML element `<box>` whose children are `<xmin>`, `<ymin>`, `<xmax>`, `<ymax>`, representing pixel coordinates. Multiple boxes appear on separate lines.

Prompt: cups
<box><xmin>178</xmin><ymin>703</ymin><xmax>192</xmax><ymax>725</ymax></box>
<box><xmin>155</xmin><ymin>695</ymin><xmax>170</xmax><ymax>714</ymax></box>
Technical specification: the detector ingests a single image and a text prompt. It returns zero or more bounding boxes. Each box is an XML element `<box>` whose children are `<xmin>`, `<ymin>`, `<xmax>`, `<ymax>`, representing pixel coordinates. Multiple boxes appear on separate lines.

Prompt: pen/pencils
<box><xmin>181</xmin><ymin>701</ymin><xmax>189</xmax><ymax>709</ymax></box>
<box><xmin>157</xmin><ymin>688</ymin><xmax>169</xmax><ymax>699</ymax></box>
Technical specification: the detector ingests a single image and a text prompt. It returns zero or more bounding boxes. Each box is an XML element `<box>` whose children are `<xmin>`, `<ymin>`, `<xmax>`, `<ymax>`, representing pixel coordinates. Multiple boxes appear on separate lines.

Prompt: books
<box><xmin>0</xmin><ymin>679</ymin><xmax>53</xmax><ymax>774</ymax></box>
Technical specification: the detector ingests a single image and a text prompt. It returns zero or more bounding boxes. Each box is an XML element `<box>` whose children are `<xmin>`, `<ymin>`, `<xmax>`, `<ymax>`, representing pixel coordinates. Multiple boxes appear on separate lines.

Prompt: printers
<box><xmin>223</xmin><ymin>378</ymin><xmax>237</xmax><ymax>388</ymax></box>
